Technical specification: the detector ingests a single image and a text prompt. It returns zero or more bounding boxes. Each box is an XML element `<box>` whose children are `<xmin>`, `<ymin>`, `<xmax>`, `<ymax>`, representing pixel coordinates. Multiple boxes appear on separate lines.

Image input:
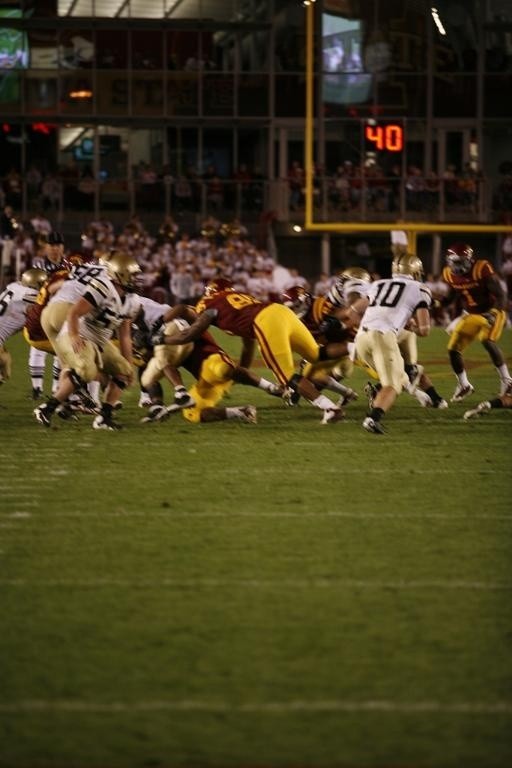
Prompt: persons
<box><xmin>1</xmin><ymin>157</ymin><xmax>490</xmax><ymax>216</ymax></box>
<box><xmin>1</xmin><ymin>206</ymin><xmax>311</xmax><ymax>305</ymax></box>
<box><xmin>0</xmin><ymin>231</ymin><xmax>512</xmax><ymax>435</ymax></box>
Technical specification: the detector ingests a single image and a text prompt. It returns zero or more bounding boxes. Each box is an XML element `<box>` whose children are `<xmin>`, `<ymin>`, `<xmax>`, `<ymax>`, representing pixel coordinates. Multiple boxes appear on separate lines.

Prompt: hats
<box><xmin>45</xmin><ymin>230</ymin><xmax>64</xmax><ymax>245</ymax></box>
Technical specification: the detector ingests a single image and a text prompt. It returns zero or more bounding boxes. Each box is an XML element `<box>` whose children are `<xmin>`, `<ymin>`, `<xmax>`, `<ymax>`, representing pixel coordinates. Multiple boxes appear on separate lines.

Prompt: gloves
<box><xmin>148</xmin><ymin>314</ymin><xmax>164</xmax><ymax>344</ymax></box>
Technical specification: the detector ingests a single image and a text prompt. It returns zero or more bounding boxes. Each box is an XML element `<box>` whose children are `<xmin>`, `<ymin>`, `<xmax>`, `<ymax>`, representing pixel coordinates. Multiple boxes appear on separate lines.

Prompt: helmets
<box><xmin>342</xmin><ymin>266</ymin><xmax>372</xmax><ymax>283</ymax></box>
<box><xmin>21</xmin><ymin>269</ymin><xmax>47</xmax><ymax>287</ymax></box>
<box><xmin>281</xmin><ymin>285</ymin><xmax>312</xmax><ymax>318</ymax></box>
<box><xmin>204</xmin><ymin>278</ymin><xmax>234</xmax><ymax>295</ymax></box>
<box><xmin>445</xmin><ymin>243</ymin><xmax>474</xmax><ymax>275</ymax></box>
<box><xmin>99</xmin><ymin>250</ymin><xmax>142</xmax><ymax>289</ymax></box>
<box><xmin>391</xmin><ymin>253</ymin><xmax>424</xmax><ymax>279</ymax></box>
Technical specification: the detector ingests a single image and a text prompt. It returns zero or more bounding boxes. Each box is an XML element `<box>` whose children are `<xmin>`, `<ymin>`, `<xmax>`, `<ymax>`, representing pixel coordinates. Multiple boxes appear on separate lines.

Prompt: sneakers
<box><xmin>243</xmin><ymin>404</ymin><xmax>257</xmax><ymax>424</ymax></box>
<box><xmin>32</xmin><ymin>386</ymin><xmax>124</xmax><ymax>431</ymax></box>
<box><xmin>138</xmin><ymin>389</ymin><xmax>195</xmax><ymax>424</ymax></box>
<box><xmin>448</xmin><ymin>384</ymin><xmax>474</xmax><ymax>402</ymax></box>
<box><xmin>464</xmin><ymin>401</ymin><xmax>491</xmax><ymax>419</ymax></box>
<box><xmin>279</xmin><ymin>365</ymin><xmax>448</xmax><ymax>434</ymax></box>
